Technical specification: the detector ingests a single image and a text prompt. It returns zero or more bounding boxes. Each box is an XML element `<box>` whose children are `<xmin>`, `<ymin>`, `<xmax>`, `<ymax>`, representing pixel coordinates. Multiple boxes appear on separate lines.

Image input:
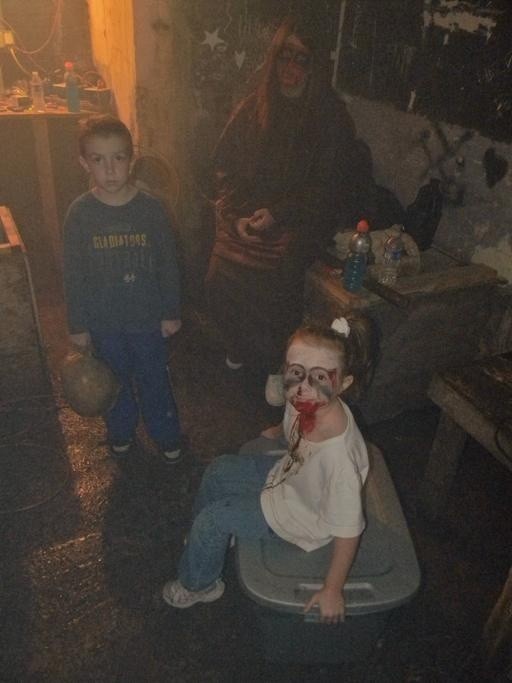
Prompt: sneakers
<box><xmin>160</xmin><ymin>446</ymin><xmax>185</xmax><ymax>465</ymax></box>
<box><xmin>162</xmin><ymin>575</ymin><xmax>226</xmax><ymax>608</ymax></box>
<box><xmin>110</xmin><ymin>435</ymin><xmax>137</xmax><ymax>458</ymax></box>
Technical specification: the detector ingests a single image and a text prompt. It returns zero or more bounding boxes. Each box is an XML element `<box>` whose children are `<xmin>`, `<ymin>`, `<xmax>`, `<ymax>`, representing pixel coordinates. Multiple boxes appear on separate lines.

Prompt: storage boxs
<box><xmin>233</xmin><ymin>436</ymin><xmax>422</xmax><ymax>665</ymax></box>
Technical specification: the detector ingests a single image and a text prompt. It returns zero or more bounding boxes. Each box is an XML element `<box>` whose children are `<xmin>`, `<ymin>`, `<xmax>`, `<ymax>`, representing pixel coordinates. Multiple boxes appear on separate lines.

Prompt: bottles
<box><xmin>342</xmin><ymin>220</ymin><xmax>371</xmax><ymax>293</ymax></box>
<box><xmin>30</xmin><ymin>71</ymin><xmax>46</xmax><ymax>111</ymax></box>
<box><xmin>65</xmin><ymin>63</ymin><xmax>81</xmax><ymax>112</ymax></box>
<box><xmin>379</xmin><ymin>232</ymin><xmax>403</xmax><ymax>286</ymax></box>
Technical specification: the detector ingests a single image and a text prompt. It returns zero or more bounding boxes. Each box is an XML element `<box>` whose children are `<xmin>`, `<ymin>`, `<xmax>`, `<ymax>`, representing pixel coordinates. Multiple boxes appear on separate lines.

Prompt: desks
<box><xmin>0</xmin><ymin>96</ymin><xmax>110</xmax><ymax>274</ymax></box>
<box><xmin>426</xmin><ymin>348</ymin><xmax>511</xmax><ymax>661</ymax></box>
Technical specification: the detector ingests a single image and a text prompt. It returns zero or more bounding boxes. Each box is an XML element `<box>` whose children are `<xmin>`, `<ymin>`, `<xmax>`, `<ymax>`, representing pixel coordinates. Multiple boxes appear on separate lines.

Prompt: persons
<box><xmin>199</xmin><ymin>9</ymin><xmax>346</xmax><ymax>409</ymax></box>
<box><xmin>57</xmin><ymin>112</ymin><xmax>184</xmax><ymax>465</ymax></box>
<box><xmin>160</xmin><ymin>308</ymin><xmax>384</xmax><ymax>626</ymax></box>
